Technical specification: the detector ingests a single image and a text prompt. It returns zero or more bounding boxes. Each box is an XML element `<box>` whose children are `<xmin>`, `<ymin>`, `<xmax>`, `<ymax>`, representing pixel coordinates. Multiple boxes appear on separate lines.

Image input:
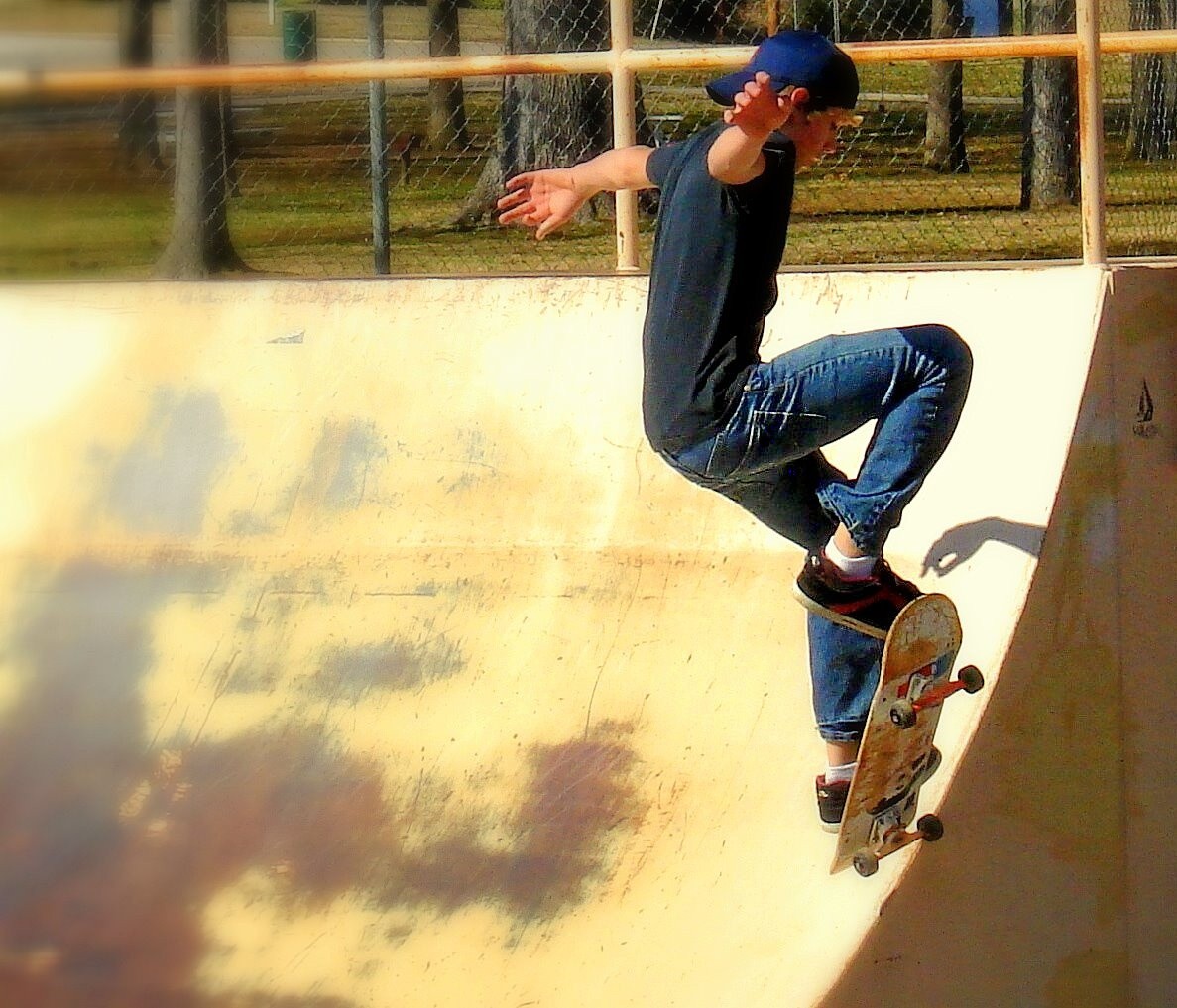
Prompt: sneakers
<box><xmin>816</xmin><ymin>743</ymin><xmax>942</xmax><ymax>834</ymax></box>
<box><xmin>792</xmin><ymin>543</ymin><xmax>925</xmax><ymax>641</ymax></box>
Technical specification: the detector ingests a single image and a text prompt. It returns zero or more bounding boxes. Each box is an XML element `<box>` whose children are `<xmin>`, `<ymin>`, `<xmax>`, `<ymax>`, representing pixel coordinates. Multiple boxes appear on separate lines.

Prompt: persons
<box><xmin>497</xmin><ymin>28</ymin><xmax>974</xmax><ymax>825</ymax></box>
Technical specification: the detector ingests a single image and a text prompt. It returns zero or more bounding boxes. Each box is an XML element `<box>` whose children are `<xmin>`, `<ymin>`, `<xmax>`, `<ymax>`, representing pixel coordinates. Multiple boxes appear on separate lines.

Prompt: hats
<box><xmin>704</xmin><ymin>29</ymin><xmax>860</xmax><ymax>110</ymax></box>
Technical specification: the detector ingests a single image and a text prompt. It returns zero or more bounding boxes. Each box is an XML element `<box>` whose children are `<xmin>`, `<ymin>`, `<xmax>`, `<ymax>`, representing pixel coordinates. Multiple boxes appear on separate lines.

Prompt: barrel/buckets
<box><xmin>282</xmin><ymin>10</ymin><xmax>316</xmax><ymax>63</ymax></box>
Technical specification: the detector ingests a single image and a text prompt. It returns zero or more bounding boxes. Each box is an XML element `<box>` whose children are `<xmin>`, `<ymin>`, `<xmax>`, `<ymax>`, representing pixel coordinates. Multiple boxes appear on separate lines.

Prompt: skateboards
<box><xmin>829</xmin><ymin>593</ymin><xmax>985</xmax><ymax>883</ymax></box>
